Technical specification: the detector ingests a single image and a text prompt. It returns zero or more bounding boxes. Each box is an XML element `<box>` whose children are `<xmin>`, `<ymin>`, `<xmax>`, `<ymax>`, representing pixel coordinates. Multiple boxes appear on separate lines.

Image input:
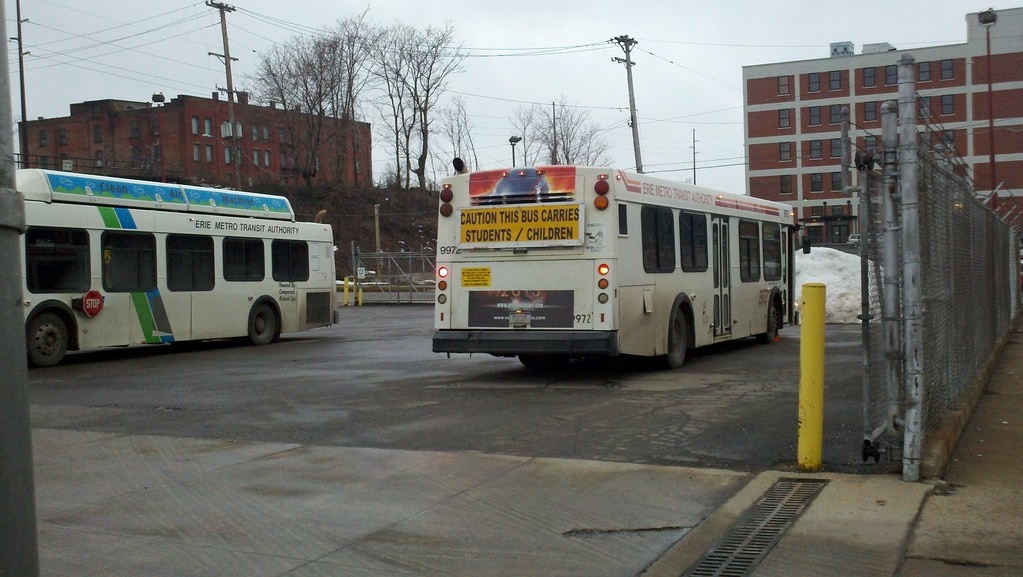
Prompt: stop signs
<box><xmin>84</xmin><ymin>289</ymin><xmax>104</xmax><ymax>318</ymax></box>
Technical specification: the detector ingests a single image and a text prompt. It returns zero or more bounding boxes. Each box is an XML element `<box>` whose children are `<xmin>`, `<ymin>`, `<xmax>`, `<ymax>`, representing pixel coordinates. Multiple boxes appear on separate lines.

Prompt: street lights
<box><xmin>975</xmin><ymin>9</ymin><xmax>1000</xmax><ymax>214</ymax></box>
<box><xmin>152</xmin><ymin>91</ymin><xmax>166</xmax><ymax>181</ymax></box>
<box><xmin>509</xmin><ymin>136</ymin><xmax>523</xmax><ymax>167</ymax></box>
<box><xmin>301</xmin><ymin>169</ymin><xmax>321</xmax><ymax>213</ymax></box>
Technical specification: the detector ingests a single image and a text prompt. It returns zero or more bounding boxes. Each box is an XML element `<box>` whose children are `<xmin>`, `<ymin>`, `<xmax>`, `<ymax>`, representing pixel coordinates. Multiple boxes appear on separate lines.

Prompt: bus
<box><xmin>11</xmin><ymin>168</ymin><xmax>341</xmax><ymax>367</ymax></box>
<box><xmin>431</xmin><ymin>164</ymin><xmax>813</xmax><ymax>370</ymax></box>
